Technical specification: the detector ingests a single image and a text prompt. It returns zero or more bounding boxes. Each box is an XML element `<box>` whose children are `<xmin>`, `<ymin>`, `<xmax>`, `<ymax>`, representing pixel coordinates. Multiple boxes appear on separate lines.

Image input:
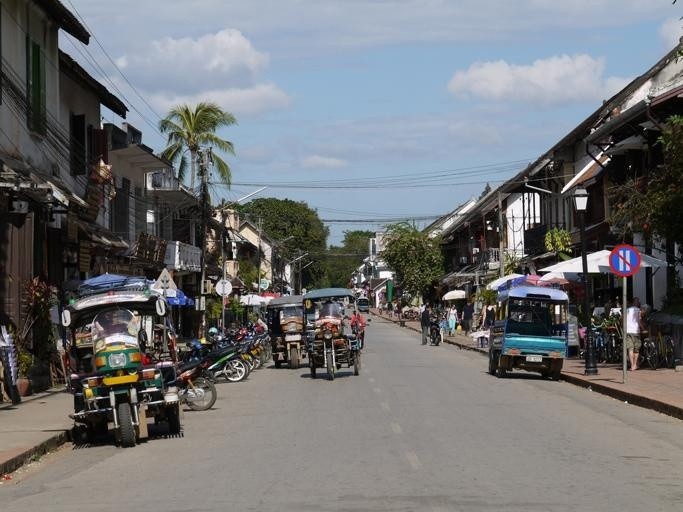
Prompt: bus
<box><xmin>486</xmin><ymin>278</ymin><xmax>570</xmax><ymax>381</ymax></box>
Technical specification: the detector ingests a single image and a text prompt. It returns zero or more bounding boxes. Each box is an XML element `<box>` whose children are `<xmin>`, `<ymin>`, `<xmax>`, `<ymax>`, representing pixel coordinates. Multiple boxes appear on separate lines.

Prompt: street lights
<box><xmin>571</xmin><ymin>188</ymin><xmax>600</xmax><ymax>375</ymax></box>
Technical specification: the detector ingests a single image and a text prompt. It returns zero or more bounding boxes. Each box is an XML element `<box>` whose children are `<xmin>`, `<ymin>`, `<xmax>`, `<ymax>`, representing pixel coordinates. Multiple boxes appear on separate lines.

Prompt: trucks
<box><xmin>357</xmin><ymin>297</ymin><xmax>369</xmax><ymax>315</ymax></box>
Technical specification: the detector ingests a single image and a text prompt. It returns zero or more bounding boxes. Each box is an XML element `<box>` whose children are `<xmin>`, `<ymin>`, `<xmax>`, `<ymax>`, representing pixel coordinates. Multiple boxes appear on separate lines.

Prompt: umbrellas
<box><xmin>442</xmin><ymin>289</ymin><xmax>469</xmax><ymax>300</ymax></box>
<box><xmin>537</xmin><ymin>250</ymin><xmax>676</xmax><ymax>276</ymax></box>
<box><xmin>487</xmin><ymin>272</ymin><xmax>585</xmax><ymax>292</ymax></box>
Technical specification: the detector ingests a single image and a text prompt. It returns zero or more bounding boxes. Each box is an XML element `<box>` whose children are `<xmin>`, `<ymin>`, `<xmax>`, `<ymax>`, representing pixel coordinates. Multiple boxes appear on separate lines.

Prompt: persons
<box><xmin>447</xmin><ymin>305</ymin><xmax>459</xmax><ymax>336</ymax></box>
<box><xmin>350</xmin><ymin>311</ymin><xmax>367</xmax><ymax>335</ymax></box>
<box><xmin>461</xmin><ymin>301</ymin><xmax>475</xmax><ymax>335</ymax></box>
<box><xmin>421</xmin><ymin>304</ymin><xmax>432</xmax><ymax>346</ymax></box>
<box><xmin>626</xmin><ymin>298</ymin><xmax>646</xmax><ymax>370</ymax></box>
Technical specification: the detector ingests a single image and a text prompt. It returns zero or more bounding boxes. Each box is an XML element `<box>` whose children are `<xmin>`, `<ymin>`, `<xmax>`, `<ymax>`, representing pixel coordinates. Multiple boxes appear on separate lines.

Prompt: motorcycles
<box><xmin>147</xmin><ymin>316</ymin><xmax>271</xmax><ymax>412</ymax></box>
<box><xmin>60</xmin><ymin>279</ymin><xmax>180</xmax><ymax>447</ymax></box>
<box><xmin>429</xmin><ymin>318</ymin><xmax>442</xmax><ymax>346</ymax></box>
<box><xmin>267</xmin><ymin>287</ymin><xmax>363</xmax><ymax>382</ymax></box>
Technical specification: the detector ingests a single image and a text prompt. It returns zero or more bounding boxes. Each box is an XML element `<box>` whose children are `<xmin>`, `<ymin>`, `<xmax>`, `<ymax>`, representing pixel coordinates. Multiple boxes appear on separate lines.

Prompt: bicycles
<box><xmin>594</xmin><ymin>312</ymin><xmax>676</xmax><ymax>369</ymax></box>
